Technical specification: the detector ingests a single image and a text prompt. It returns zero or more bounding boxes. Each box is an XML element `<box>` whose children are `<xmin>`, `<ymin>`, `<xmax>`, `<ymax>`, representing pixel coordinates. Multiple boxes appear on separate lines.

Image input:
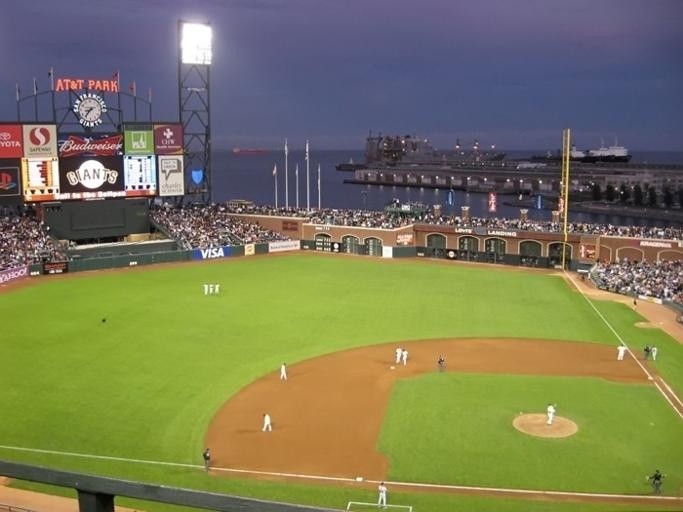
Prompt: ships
<box><xmin>361</xmin><ymin>129</ymin><xmax>508</xmax><ymax>169</ymax></box>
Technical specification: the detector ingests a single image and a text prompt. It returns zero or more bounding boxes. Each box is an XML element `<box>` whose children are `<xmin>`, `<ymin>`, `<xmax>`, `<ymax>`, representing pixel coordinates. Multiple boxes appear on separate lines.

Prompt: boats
<box><xmin>551</xmin><ymin>144</ymin><xmax>632</xmax><ymax>164</ymax></box>
<box><xmin>232</xmin><ymin>146</ymin><xmax>272</xmax><ymax>156</ymax></box>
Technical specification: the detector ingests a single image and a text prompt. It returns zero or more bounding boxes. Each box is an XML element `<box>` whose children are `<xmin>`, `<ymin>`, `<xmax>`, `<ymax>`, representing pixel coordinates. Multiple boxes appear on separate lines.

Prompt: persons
<box><xmin>378</xmin><ymin>483</ymin><xmax>387</xmax><ymax>509</ymax></box>
<box><xmin>402</xmin><ymin>349</ymin><xmax>409</xmax><ymax>366</ymax></box>
<box><xmin>424</xmin><ymin>211</ymin><xmax>683</xmax><ymax>242</ymax></box>
<box><xmin>588</xmin><ymin>259</ymin><xmax>683</xmax><ymax>309</ymax></box>
<box><xmin>617</xmin><ymin>344</ymin><xmax>626</xmax><ymax>361</ymax></box>
<box><xmin>262</xmin><ymin>413</ymin><xmax>271</xmax><ymax>432</ymax></box>
<box><xmin>395</xmin><ymin>346</ymin><xmax>402</xmax><ymax>364</ymax></box>
<box><xmin>644</xmin><ymin>344</ymin><xmax>652</xmax><ymax>359</ymax></box>
<box><xmin>203</xmin><ymin>448</ymin><xmax>211</xmax><ymax>472</ymax></box>
<box><xmin>646</xmin><ymin>470</ymin><xmax>667</xmax><ymax>495</ymax></box>
<box><xmin>546</xmin><ymin>403</ymin><xmax>555</xmax><ymax>424</ymax></box>
<box><xmin>652</xmin><ymin>346</ymin><xmax>658</xmax><ymax>360</ymax></box>
<box><xmin>150</xmin><ymin>196</ymin><xmax>422</xmax><ymax>249</ymax></box>
<box><xmin>280</xmin><ymin>362</ymin><xmax>287</xmax><ymax>382</ymax></box>
<box><xmin>0</xmin><ymin>213</ymin><xmax>73</xmax><ymax>271</ymax></box>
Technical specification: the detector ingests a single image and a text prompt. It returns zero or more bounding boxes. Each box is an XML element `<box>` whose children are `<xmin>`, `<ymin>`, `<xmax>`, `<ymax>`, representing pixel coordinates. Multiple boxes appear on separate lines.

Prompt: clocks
<box><xmin>72</xmin><ymin>92</ymin><xmax>107</xmax><ymax>125</ymax></box>
